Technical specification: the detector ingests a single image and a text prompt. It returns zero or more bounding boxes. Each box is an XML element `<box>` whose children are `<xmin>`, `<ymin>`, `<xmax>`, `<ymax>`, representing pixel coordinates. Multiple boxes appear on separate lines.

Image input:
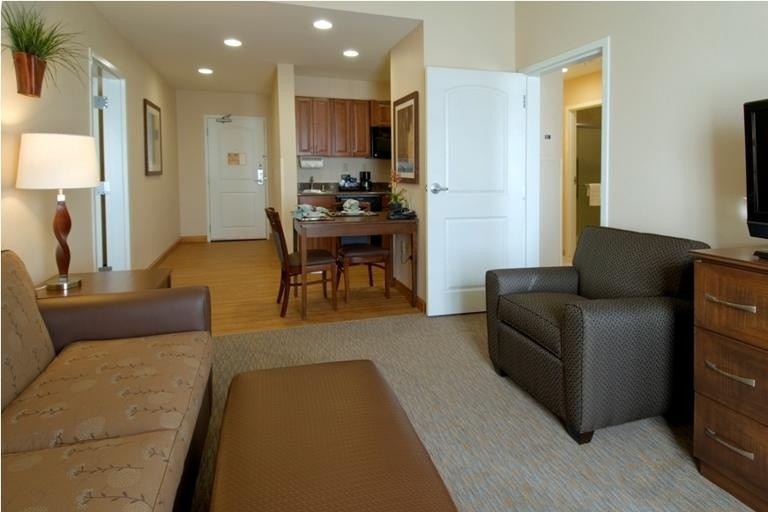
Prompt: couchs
<box><xmin>1</xmin><ymin>249</ymin><xmax>216</xmax><ymax>512</ymax></box>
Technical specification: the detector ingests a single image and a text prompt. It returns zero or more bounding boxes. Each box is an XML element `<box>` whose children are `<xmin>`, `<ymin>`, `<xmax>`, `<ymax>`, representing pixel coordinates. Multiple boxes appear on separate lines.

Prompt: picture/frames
<box><xmin>393</xmin><ymin>91</ymin><xmax>418</xmax><ymax>183</ymax></box>
<box><xmin>144</xmin><ymin>98</ymin><xmax>163</xmax><ymax>176</ymax></box>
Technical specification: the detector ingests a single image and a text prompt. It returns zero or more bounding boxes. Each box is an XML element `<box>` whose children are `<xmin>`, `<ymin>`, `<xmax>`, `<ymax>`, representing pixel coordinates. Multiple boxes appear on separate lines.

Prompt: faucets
<box><xmin>310</xmin><ymin>176</ymin><xmax>314</xmax><ymax>189</ymax></box>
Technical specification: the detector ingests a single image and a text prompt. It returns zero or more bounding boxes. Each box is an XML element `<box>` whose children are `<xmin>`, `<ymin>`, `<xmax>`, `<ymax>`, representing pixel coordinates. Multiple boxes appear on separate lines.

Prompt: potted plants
<box><xmin>1</xmin><ymin>2</ymin><xmax>90</xmax><ymax>98</ymax></box>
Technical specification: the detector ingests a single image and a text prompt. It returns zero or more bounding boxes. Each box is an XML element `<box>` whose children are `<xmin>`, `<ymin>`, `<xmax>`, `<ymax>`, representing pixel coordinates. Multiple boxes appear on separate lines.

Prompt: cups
<box><xmin>337</xmin><ymin>175</ymin><xmax>359</xmax><ymax>190</ymax></box>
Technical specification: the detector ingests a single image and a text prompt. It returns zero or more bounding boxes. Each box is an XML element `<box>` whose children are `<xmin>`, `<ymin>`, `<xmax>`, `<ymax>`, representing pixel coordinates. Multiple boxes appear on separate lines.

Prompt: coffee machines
<box><xmin>359</xmin><ymin>170</ymin><xmax>372</xmax><ymax>193</ymax></box>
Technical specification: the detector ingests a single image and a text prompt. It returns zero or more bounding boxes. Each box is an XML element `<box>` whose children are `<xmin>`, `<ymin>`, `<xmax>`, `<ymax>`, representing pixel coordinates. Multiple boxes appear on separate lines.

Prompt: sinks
<box><xmin>303</xmin><ymin>189</ymin><xmax>322</xmax><ymax>193</ymax></box>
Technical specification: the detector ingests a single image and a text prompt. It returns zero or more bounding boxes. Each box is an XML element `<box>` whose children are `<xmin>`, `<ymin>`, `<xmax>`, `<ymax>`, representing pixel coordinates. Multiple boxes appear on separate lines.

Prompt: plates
<box><xmin>288</xmin><ymin>202</ymin><xmax>368</xmax><ymax>223</ymax></box>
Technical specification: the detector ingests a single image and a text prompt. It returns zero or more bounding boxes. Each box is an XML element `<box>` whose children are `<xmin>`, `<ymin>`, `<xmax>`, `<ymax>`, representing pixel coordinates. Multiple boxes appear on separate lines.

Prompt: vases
<box><xmin>387</xmin><ymin>201</ymin><xmax>401</xmax><ymax>210</ymax></box>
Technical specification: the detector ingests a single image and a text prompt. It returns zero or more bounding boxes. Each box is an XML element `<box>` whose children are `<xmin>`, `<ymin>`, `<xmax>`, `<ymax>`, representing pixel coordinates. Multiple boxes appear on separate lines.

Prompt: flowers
<box><xmin>384</xmin><ymin>170</ymin><xmax>408</xmax><ymax>203</ymax></box>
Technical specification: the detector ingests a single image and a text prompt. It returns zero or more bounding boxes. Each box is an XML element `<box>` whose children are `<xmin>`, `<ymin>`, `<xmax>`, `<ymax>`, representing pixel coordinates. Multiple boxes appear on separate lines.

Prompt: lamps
<box><xmin>15</xmin><ymin>133</ymin><xmax>100</xmax><ymax>292</ymax></box>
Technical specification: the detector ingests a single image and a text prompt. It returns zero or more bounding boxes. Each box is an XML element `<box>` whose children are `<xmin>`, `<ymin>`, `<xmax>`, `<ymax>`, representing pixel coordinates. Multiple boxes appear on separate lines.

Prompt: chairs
<box><xmin>485</xmin><ymin>224</ymin><xmax>711</xmax><ymax>445</ymax></box>
<box><xmin>334</xmin><ymin>202</ymin><xmax>390</xmax><ymax>304</ymax></box>
<box><xmin>265</xmin><ymin>207</ymin><xmax>338</xmax><ymax>318</ymax></box>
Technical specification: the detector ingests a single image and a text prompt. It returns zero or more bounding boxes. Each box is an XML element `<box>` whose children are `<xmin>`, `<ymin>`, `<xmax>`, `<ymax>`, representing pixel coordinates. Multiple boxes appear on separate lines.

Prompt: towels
<box><xmin>586</xmin><ymin>182</ymin><xmax>600</xmax><ymax>207</ymax></box>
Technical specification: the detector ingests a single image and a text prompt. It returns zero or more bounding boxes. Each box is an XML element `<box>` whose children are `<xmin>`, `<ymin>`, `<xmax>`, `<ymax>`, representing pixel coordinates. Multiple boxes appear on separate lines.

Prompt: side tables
<box><xmin>33</xmin><ymin>268</ymin><xmax>171</xmax><ymax>297</ymax></box>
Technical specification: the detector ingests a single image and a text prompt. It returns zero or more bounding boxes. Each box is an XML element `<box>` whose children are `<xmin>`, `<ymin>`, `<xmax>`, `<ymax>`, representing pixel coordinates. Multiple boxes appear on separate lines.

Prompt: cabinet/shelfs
<box><xmin>295</xmin><ymin>96</ymin><xmax>390</xmax><ymax>159</ymax></box>
<box><xmin>685</xmin><ymin>244</ymin><xmax>768</xmax><ymax>512</ymax></box>
<box><xmin>299</xmin><ymin>196</ymin><xmax>333</xmax><ymax>253</ymax></box>
<box><xmin>381</xmin><ymin>196</ymin><xmax>392</xmax><ymax>249</ymax></box>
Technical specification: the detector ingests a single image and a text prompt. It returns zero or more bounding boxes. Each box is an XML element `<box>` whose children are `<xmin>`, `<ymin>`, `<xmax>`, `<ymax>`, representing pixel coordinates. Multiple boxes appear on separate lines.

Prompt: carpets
<box><xmin>191</xmin><ymin>310</ymin><xmax>766</xmax><ymax>511</ymax></box>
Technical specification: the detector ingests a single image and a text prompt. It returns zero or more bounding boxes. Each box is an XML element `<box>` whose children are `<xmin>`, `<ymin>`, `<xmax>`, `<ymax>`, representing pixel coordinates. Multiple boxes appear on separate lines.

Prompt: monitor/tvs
<box><xmin>743</xmin><ymin>98</ymin><xmax>768</xmax><ymax>259</ymax></box>
